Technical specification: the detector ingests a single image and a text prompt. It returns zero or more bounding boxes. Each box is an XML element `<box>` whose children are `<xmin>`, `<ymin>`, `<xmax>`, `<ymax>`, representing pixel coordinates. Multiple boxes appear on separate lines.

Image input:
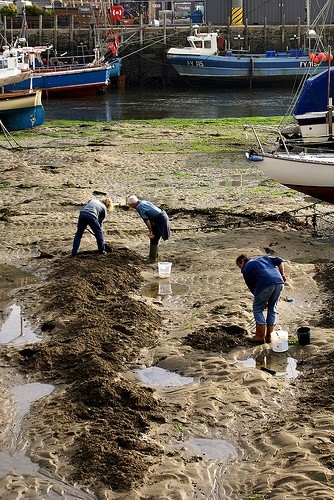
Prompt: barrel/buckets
<box><xmin>270</xmin><ymin>329</ymin><xmax>289</xmax><ymax>353</ymax></box>
<box><xmin>158</xmin><ymin>282</ymin><xmax>173</xmax><ymax>295</ymax></box>
<box><xmin>295</xmin><ymin>325</ymin><xmax>311</xmax><ymax>347</ymax></box>
<box><xmin>289</xmin><ymin>49</ymin><xmax>304</xmax><ymax>58</ymax></box>
<box><xmin>157</xmin><ymin>260</ymin><xmax>173</xmax><ymax>279</ymax></box>
<box><xmin>267</xmin><ymin>51</ymin><xmax>276</xmax><ymax>57</ymax></box>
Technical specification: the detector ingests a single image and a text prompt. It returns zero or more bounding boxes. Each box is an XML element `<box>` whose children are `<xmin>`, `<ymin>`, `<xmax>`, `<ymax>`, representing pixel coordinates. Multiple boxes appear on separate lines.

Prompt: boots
<box><xmin>264</xmin><ymin>325</ymin><xmax>273</xmax><ymax>342</ymax></box>
<box><xmin>248</xmin><ymin>325</ymin><xmax>265</xmax><ymax>344</ymax></box>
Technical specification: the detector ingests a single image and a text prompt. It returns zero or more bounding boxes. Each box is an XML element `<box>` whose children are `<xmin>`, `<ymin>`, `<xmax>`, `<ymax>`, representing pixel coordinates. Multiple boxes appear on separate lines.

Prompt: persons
<box><xmin>70</xmin><ymin>197</ymin><xmax>112</xmax><ymax>254</ymax></box>
<box><xmin>127</xmin><ymin>196</ymin><xmax>171</xmax><ymax>263</ymax></box>
<box><xmin>235</xmin><ymin>252</ymin><xmax>287</xmax><ymax>345</ymax></box>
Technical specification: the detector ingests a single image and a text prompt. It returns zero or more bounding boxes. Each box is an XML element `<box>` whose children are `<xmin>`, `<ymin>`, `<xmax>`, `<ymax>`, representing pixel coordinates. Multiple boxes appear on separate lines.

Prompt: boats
<box><xmin>3</xmin><ymin>6</ymin><xmax>125</xmax><ymax>77</ymax></box>
<box><xmin>0</xmin><ymin>14</ymin><xmax>110</xmax><ymax>100</ymax></box>
<box><xmin>0</xmin><ymin>76</ymin><xmax>46</xmax><ymax>133</ymax></box>
<box><xmin>249</xmin><ymin>0</ymin><xmax>334</xmax><ymax>203</ymax></box>
<box><xmin>167</xmin><ymin>24</ymin><xmax>333</xmax><ymax>87</ymax></box>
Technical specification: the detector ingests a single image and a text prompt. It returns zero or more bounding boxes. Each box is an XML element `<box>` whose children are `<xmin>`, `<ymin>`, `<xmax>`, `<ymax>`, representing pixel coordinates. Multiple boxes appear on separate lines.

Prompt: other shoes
<box><xmin>144</xmin><ymin>258</ymin><xmax>157</xmax><ymax>263</ymax></box>
